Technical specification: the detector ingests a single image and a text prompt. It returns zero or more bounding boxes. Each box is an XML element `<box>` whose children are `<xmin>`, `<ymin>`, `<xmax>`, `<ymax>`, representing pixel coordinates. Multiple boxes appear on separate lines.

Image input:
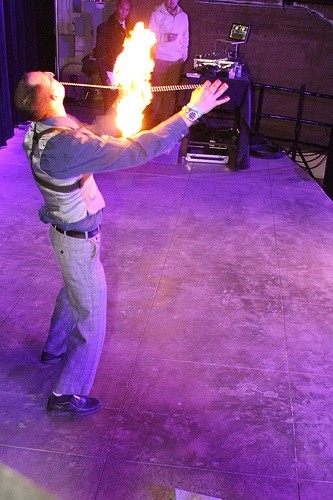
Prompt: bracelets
<box><xmin>187</xmin><ymin>103</ymin><xmax>203</xmax><ymax>118</ymax></box>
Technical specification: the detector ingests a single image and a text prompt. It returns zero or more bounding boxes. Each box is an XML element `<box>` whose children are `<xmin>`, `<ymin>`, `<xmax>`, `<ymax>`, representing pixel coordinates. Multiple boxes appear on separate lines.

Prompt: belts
<box><xmin>53</xmin><ymin>224</ymin><xmax>101</xmax><ymax>240</ymax></box>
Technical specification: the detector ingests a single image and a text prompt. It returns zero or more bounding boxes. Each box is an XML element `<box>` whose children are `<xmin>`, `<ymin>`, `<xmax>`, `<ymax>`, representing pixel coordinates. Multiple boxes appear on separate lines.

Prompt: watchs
<box><xmin>181</xmin><ymin>106</ymin><xmax>199</xmax><ymax>126</ymax></box>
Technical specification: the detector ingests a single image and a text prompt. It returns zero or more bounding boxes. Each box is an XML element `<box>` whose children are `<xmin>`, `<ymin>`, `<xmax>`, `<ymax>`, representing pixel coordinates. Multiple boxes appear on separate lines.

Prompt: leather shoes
<box><xmin>48</xmin><ymin>394</ymin><xmax>100</xmax><ymax>416</ymax></box>
<box><xmin>41</xmin><ymin>350</ymin><xmax>66</xmax><ymax>364</ymax></box>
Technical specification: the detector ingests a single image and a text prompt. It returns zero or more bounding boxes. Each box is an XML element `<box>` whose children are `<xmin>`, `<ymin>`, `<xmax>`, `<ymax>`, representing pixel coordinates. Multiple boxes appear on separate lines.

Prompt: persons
<box><xmin>16</xmin><ymin>71</ymin><xmax>231</xmax><ymax>417</ymax></box>
<box><xmin>149</xmin><ymin>0</ymin><xmax>189</xmax><ymax>124</ymax></box>
<box><xmin>96</xmin><ymin>0</ymin><xmax>132</xmax><ymax>113</ymax></box>
